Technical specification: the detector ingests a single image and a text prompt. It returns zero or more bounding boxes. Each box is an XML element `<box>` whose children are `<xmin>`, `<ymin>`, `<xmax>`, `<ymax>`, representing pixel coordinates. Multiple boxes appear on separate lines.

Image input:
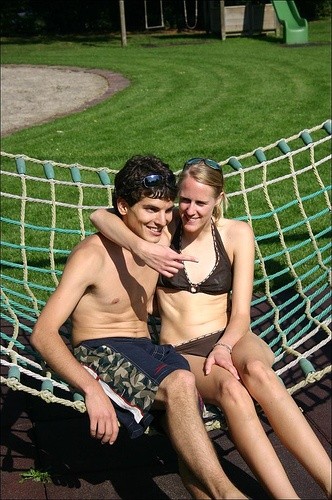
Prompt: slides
<box><xmin>271</xmin><ymin>0</ymin><xmax>309</xmax><ymax>44</ymax></box>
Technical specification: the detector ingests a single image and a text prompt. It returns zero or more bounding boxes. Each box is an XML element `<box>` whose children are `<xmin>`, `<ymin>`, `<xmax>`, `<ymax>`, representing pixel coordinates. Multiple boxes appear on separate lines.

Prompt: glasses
<box><xmin>121</xmin><ymin>173</ymin><xmax>176</xmax><ymax>198</ymax></box>
<box><xmin>183</xmin><ymin>157</ymin><xmax>223</xmax><ymax>179</ymax></box>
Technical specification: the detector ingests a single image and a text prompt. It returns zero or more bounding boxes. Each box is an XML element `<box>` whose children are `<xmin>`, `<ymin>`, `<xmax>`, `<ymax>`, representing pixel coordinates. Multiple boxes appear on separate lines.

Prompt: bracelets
<box><xmin>213</xmin><ymin>343</ymin><xmax>233</xmax><ymax>354</ymax></box>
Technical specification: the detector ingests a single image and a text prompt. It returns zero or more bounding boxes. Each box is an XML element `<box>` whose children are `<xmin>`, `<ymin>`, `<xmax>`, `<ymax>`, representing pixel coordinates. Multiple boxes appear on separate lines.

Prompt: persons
<box><xmin>89</xmin><ymin>158</ymin><xmax>331</xmax><ymax>500</ymax></box>
<box><xmin>29</xmin><ymin>152</ymin><xmax>253</xmax><ymax>500</ymax></box>
